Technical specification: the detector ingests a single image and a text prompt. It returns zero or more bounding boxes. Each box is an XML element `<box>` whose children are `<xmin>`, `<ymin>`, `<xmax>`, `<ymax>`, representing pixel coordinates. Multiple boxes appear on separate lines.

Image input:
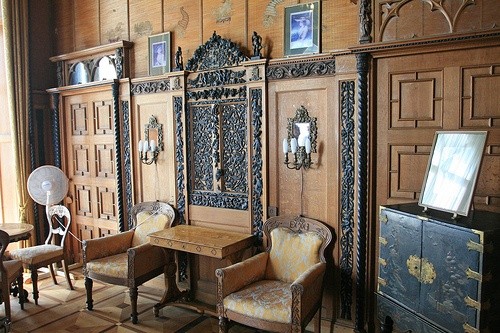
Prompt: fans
<box><xmin>24</xmin><ymin>165</ymin><xmax>82</xmax><ymax>284</ymax></box>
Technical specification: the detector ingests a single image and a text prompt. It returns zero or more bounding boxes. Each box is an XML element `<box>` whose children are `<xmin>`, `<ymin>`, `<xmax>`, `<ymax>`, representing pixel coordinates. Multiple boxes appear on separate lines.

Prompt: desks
<box><xmin>0</xmin><ymin>222</ymin><xmax>35</xmax><ymax>303</ymax></box>
<box><xmin>146</xmin><ymin>224</ymin><xmax>254</xmax><ymax>320</ymax></box>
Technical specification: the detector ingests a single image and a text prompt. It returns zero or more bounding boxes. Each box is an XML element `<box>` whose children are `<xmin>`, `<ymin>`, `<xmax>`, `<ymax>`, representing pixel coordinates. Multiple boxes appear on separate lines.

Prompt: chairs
<box><xmin>82</xmin><ymin>200</ymin><xmax>176</xmax><ymax>324</ymax></box>
<box><xmin>216</xmin><ymin>215</ymin><xmax>333</xmax><ymax>333</ymax></box>
<box><xmin>9</xmin><ymin>204</ymin><xmax>73</xmax><ymax>305</ymax></box>
<box><xmin>0</xmin><ymin>230</ymin><xmax>25</xmax><ymax>333</ymax></box>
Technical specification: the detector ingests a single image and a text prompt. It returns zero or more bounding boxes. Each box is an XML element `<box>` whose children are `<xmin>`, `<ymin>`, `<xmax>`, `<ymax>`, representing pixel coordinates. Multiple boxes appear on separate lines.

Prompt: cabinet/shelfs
<box><xmin>377</xmin><ymin>202</ymin><xmax>500</xmax><ymax>333</ymax></box>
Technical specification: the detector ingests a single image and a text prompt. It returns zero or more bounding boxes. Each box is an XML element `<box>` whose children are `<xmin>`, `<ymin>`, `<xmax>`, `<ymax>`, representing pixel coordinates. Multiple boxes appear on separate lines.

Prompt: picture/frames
<box><xmin>417</xmin><ymin>130</ymin><xmax>489</xmax><ymax>220</ymax></box>
<box><xmin>282</xmin><ymin>1</ymin><xmax>321</xmax><ymax>57</ymax></box>
<box><xmin>147</xmin><ymin>30</ymin><xmax>171</xmax><ymax>74</ymax></box>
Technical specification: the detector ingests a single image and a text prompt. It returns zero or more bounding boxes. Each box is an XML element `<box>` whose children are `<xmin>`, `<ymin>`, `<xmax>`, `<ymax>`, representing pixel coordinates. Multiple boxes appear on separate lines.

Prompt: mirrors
<box><xmin>143</xmin><ymin>116</ymin><xmax>164</xmax><ymax>151</ymax></box>
<box><xmin>286</xmin><ymin>105</ymin><xmax>318</xmax><ymax>153</ymax></box>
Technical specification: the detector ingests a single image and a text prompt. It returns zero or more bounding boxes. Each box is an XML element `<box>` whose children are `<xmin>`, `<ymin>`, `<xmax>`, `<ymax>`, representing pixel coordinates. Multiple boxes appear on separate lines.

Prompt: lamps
<box><xmin>137</xmin><ymin>139</ymin><xmax>159</xmax><ymax>165</ymax></box>
<box><xmin>283</xmin><ymin>137</ymin><xmax>312</xmax><ymax>170</ymax></box>
